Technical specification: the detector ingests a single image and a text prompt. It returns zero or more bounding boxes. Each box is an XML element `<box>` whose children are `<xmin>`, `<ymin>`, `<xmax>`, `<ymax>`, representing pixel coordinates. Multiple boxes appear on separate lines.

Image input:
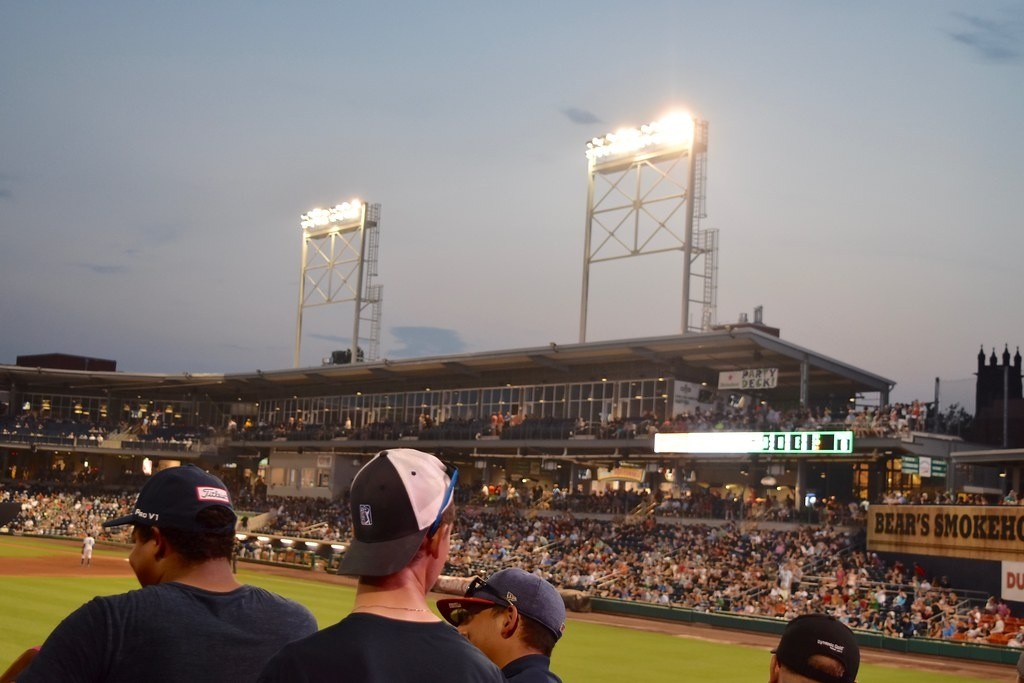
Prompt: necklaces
<box><xmin>352</xmin><ymin>604</ymin><xmax>432</xmax><ymax>612</ymax></box>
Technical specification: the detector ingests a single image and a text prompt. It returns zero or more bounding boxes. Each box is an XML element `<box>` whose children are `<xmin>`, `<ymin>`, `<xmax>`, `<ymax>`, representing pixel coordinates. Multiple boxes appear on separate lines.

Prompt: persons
<box><xmin>14</xmin><ymin>462</ymin><xmax>319</xmax><ymax>683</ymax></box>
<box><xmin>80</xmin><ymin>533</ymin><xmax>95</xmax><ymax>567</ymax></box>
<box><xmin>255</xmin><ymin>448</ymin><xmax>503</xmax><ymax>683</ymax></box>
<box><xmin>436</xmin><ymin>568</ymin><xmax>565</xmax><ymax>683</ymax></box>
<box><xmin>0</xmin><ymin>390</ymin><xmax>1024</xmax><ymax>650</ymax></box>
<box><xmin>768</xmin><ymin>613</ymin><xmax>860</xmax><ymax>683</ymax></box>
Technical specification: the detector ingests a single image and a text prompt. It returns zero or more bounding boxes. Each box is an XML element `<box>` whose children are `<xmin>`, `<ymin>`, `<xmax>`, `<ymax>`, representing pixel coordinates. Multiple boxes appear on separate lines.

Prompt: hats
<box><xmin>1017</xmin><ymin>651</ymin><xmax>1024</xmax><ymax>676</ymax></box>
<box><xmin>771</xmin><ymin>614</ymin><xmax>860</xmax><ymax>683</ymax></box>
<box><xmin>437</xmin><ymin>568</ymin><xmax>565</xmax><ymax>638</ymax></box>
<box><xmin>326</xmin><ymin>449</ymin><xmax>453</xmax><ymax>576</ymax></box>
<box><xmin>101</xmin><ymin>463</ymin><xmax>237</xmax><ymax>535</ymax></box>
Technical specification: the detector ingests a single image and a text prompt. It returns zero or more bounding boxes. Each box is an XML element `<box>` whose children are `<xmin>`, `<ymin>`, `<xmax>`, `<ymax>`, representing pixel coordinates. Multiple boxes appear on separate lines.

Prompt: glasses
<box><xmin>464</xmin><ymin>576</ymin><xmax>513</xmax><ymax>605</ymax></box>
<box><xmin>427</xmin><ymin>460</ymin><xmax>460</xmax><ymax>540</ymax></box>
<box><xmin>450</xmin><ymin>604</ymin><xmax>490</xmax><ymax>626</ymax></box>
